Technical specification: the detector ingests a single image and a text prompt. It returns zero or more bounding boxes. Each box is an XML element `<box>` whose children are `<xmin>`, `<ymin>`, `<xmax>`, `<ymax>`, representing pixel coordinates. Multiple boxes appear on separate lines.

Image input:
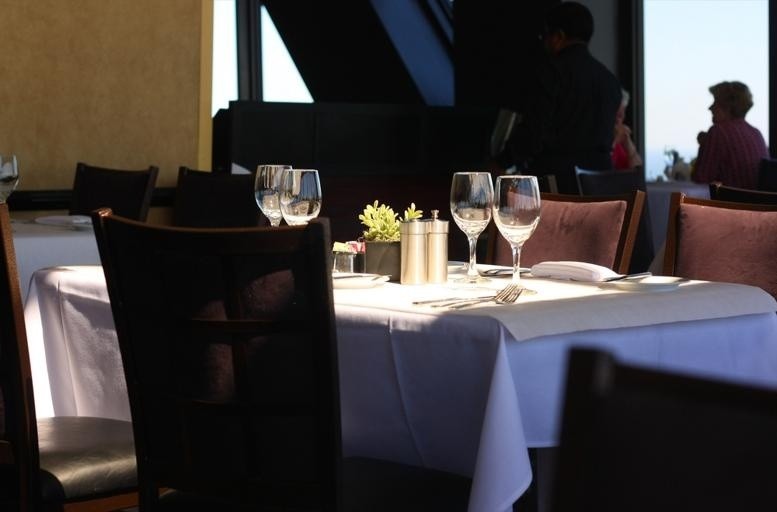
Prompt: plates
<box><xmin>598</xmin><ymin>273</ymin><xmax>688</xmax><ymax>295</ymax></box>
<box><xmin>332</xmin><ymin>269</ymin><xmax>389</xmax><ymax>290</ymax></box>
<box><xmin>463</xmin><ymin>263</ymin><xmax>532</xmax><ymax>277</ymax></box>
<box><xmin>36</xmin><ymin>215</ymin><xmax>92</xmax><ymax>225</ymax></box>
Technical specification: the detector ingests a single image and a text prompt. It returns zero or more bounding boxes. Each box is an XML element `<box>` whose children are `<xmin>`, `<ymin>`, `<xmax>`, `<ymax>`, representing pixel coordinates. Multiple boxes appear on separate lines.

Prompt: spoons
<box><xmin>481</xmin><ymin>263</ymin><xmax>532</xmax><ymax>275</ymax></box>
<box><xmin>412</xmin><ymin>289</ymin><xmax>462</xmax><ymax>302</ymax></box>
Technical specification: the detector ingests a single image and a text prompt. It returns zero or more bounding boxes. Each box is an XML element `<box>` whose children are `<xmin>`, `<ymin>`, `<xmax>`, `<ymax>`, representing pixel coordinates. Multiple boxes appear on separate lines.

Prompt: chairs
<box><xmin>68</xmin><ymin>163</ymin><xmax>159</xmax><ymax>223</ymax></box>
<box><xmin>0</xmin><ymin>204</ymin><xmax>68</xmax><ymax>511</ymax></box>
<box><xmin>758</xmin><ymin>157</ymin><xmax>777</xmax><ymax>190</ymax></box>
<box><xmin>173</xmin><ymin>167</ymin><xmax>223</xmax><ymax>214</ymax></box>
<box><xmin>710</xmin><ymin>180</ymin><xmax>776</xmax><ymax>205</ymax></box>
<box><xmin>485</xmin><ymin>187</ymin><xmax>646</xmax><ymax>276</ymax></box>
<box><xmin>92</xmin><ymin>206</ymin><xmax>466</xmax><ymax>511</ymax></box>
<box><xmin>553</xmin><ymin>345</ymin><xmax>777</xmax><ymax>510</ymax></box>
<box><xmin>574</xmin><ymin>165</ymin><xmax>655</xmax><ymax>273</ymax></box>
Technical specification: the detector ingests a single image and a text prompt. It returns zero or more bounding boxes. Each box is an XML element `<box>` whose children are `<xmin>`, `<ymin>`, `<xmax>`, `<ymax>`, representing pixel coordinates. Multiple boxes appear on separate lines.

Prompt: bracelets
<box><xmin>628</xmin><ymin>151</ymin><xmax>638</xmax><ymax>161</ymax></box>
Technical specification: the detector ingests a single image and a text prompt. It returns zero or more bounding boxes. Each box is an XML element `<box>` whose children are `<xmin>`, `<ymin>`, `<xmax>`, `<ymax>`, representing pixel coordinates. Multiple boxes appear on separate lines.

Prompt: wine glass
<box><xmin>281</xmin><ymin>171</ymin><xmax>320</xmax><ymax>226</ymax></box>
<box><xmin>450</xmin><ymin>173</ymin><xmax>497</xmax><ymax>293</ymax></box>
<box><xmin>496</xmin><ymin>175</ymin><xmax>542</xmax><ymax>292</ymax></box>
<box><xmin>255</xmin><ymin>162</ymin><xmax>287</xmax><ymax>228</ymax></box>
<box><xmin>0</xmin><ymin>155</ymin><xmax>21</xmax><ymax>213</ymax></box>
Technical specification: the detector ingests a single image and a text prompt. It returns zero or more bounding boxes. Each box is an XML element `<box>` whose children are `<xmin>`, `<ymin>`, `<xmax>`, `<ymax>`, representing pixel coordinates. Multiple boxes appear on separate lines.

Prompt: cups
<box><xmin>333</xmin><ymin>252</ymin><xmax>364</xmax><ymax>277</ymax></box>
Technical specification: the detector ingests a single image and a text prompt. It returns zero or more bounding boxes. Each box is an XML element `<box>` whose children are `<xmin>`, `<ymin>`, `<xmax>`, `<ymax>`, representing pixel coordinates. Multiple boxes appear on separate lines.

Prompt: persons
<box><xmin>508</xmin><ymin>3</ymin><xmax>622</xmax><ymax>194</ymax></box>
<box><xmin>610</xmin><ymin>89</ymin><xmax>644</xmax><ymax>171</ymax></box>
<box><xmin>690</xmin><ymin>82</ymin><xmax>770</xmax><ymax>193</ymax></box>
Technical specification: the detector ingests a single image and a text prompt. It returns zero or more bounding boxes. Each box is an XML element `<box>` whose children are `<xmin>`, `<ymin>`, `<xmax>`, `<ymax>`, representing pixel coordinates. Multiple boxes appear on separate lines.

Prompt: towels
<box><xmin>34</xmin><ymin>215</ymin><xmax>93</xmax><ymax>227</ymax></box>
<box><xmin>531</xmin><ymin>261</ymin><xmax>621</xmax><ymax>283</ymax></box>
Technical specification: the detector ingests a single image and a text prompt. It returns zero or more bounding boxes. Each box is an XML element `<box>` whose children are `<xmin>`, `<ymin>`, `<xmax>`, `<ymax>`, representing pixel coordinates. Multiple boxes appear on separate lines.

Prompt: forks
<box><xmin>452</xmin><ymin>290</ymin><xmax>524</xmax><ymax>310</ymax></box>
<box><xmin>434</xmin><ymin>285</ymin><xmax>518</xmax><ymax>308</ymax></box>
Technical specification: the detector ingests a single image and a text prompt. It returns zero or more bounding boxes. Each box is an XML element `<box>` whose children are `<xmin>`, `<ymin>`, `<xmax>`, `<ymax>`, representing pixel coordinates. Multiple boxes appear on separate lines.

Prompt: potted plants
<box><xmin>359</xmin><ymin>199</ymin><xmax>423</xmax><ymax>279</ymax></box>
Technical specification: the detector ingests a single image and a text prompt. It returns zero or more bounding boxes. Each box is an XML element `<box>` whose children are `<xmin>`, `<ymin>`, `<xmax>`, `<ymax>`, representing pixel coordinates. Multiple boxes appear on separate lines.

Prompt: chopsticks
<box><xmin>602</xmin><ymin>267</ymin><xmax>654</xmax><ymax>289</ymax></box>
<box><xmin>331</xmin><ymin>273</ymin><xmax>393</xmax><ymax>280</ymax></box>
